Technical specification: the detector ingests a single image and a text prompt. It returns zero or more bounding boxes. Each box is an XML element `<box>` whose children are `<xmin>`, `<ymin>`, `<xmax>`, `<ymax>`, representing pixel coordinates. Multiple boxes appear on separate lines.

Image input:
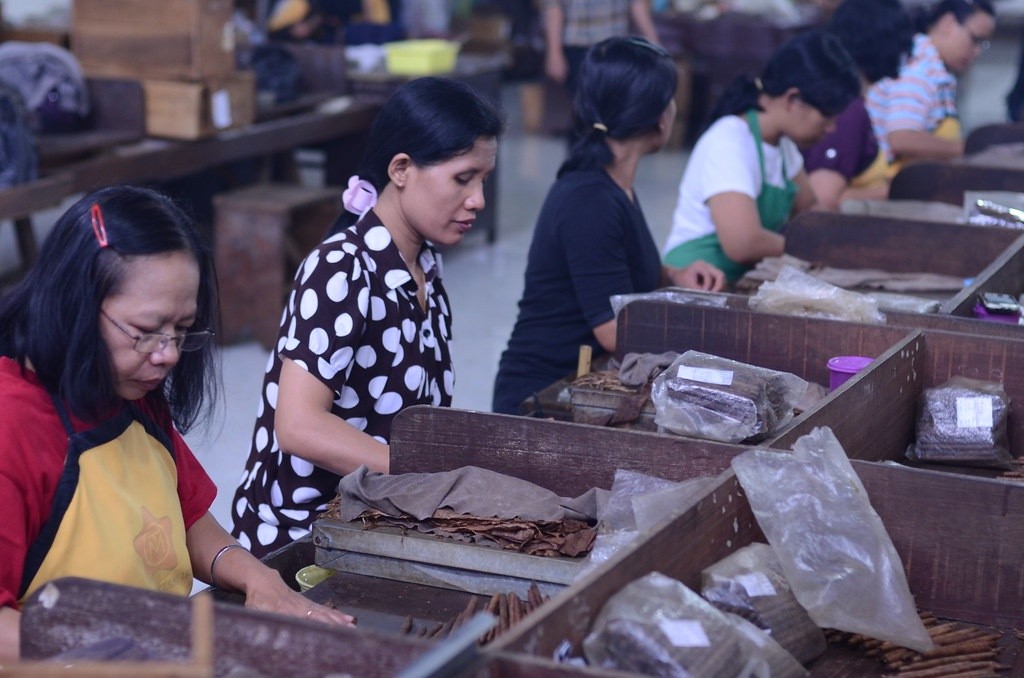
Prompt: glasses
<box><xmin>100</xmin><ymin>307</ymin><xmax>216</xmax><ymax>353</ymax></box>
<box><xmin>963</xmin><ymin>21</ymin><xmax>992</xmax><ymax>48</ymax></box>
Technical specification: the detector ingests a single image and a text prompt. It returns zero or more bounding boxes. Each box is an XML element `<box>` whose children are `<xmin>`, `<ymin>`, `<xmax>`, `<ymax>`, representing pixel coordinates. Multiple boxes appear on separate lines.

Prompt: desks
<box><xmin>0</xmin><ymin>63</ymin><xmax>507</xmax><ymax>338</ymax></box>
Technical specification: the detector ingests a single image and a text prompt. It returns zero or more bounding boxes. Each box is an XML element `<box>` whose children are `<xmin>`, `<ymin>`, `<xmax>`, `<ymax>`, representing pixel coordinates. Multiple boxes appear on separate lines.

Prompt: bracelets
<box><xmin>210</xmin><ymin>545</ymin><xmax>247</xmax><ymax>585</ymax></box>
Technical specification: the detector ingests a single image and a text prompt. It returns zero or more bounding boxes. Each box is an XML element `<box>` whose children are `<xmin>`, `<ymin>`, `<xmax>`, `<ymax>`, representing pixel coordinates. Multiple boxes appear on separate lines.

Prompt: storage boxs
<box><xmin>139</xmin><ymin>70</ymin><xmax>258</xmax><ymax>143</ymax></box>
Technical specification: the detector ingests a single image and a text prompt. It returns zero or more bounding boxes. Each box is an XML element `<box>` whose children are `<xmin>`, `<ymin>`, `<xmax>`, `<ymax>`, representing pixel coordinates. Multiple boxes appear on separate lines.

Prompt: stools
<box><xmin>215</xmin><ymin>191</ymin><xmax>348</xmax><ymax>348</ymax></box>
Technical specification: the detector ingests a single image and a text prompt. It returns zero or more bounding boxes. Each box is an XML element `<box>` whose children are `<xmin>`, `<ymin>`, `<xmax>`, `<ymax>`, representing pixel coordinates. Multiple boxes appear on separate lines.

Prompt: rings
<box><xmin>306</xmin><ymin>610</ymin><xmax>313</xmax><ymax>618</ymax></box>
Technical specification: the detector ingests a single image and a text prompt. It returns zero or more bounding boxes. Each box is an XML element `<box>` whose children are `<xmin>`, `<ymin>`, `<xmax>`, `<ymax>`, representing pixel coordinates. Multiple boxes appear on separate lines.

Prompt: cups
<box><xmin>973</xmin><ymin>303</ymin><xmax>1023</xmax><ymax>324</ymax></box>
<box><xmin>294</xmin><ymin>565</ymin><xmax>334</xmax><ymax>592</ymax></box>
<box><xmin>826</xmin><ymin>355</ymin><xmax>877</xmax><ymax>394</ymax></box>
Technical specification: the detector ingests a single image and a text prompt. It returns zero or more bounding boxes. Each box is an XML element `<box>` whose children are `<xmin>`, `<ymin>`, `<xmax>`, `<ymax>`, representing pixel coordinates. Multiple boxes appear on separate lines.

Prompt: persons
<box><xmin>0</xmin><ymin>183</ymin><xmax>358</xmax><ymax>661</ymax></box>
<box><xmin>341</xmin><ymin>0</ymin><xmax>404</xmax><ymax>48</ymax></box>
<box><xmin>803</xmin><ymin>0</ymin><xmax>916</xmax><ymax>209</ymax></box>
<box><xmin>221</xmin><ymin>0</ymin><xmax>299</xmax><ymax>104</ymax></box>
<box><xmin>491</xmin><ymin>35</ymin><xmax>724</xmax><ymax>416</ymax></box>
<box><xmin>665</xmin><ymin>28</ymin><xmax>862</xmax><ymax>291</ymax></box>
<box><xmin>540</xmin><ymin>0</ymin><xmax>660</xmax><ymax>91</ymax></box>
<box><xmin>865</xmin><ymin>0</ymin><xmax>998</xmax><ymax>163</ymax></box>
<box><xmin>230</xmin><ymin>78</ymin><xmax>504</xmax><ymax>557</ymax></box>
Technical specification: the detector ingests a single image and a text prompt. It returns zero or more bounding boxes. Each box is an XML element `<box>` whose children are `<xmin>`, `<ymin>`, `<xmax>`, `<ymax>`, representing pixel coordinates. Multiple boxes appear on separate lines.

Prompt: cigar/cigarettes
<box><xmin>589</xmin><ymin>540</ymin><xmax>1010</xmax><ymax>677</ymax></box>
<box><xmin>916</xmin><ymin>388</ymin><xmax>1024</xmax><ymax>482</ymax></box>
<box><xmin>398</xmin><ymin>582</ymin><xmax>550</xmax><ymax>654</ymax></box>
<box><xmin>663</xmin><ymin>353</ymin><xmax>789</xmax><ymax>438</ymax></box>
